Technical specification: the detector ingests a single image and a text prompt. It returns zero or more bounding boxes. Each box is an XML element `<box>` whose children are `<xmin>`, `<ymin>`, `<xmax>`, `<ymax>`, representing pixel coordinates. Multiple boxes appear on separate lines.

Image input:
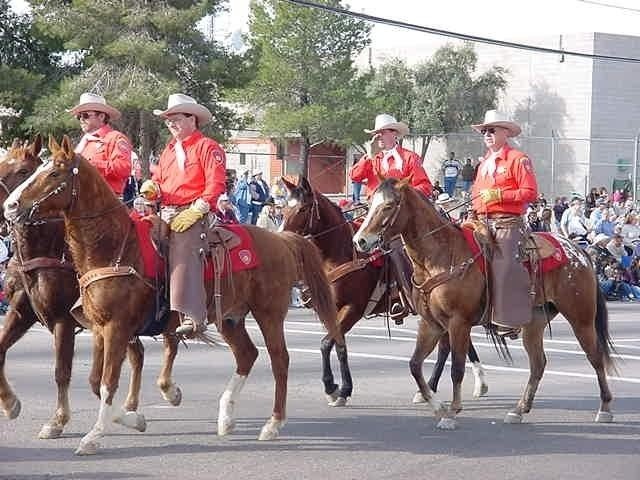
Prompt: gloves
<box><xmin>140</xmin><ymin>180</ymin><xmax>157</xmax><ymax>200</ymax></box>
<box><xmin>479</xmin><ymin>188</ymin><xmax>503</xmax><ymax>204</ymax></box>
<box><xmin>367</xmin><ymin>135</ymin><xmax>385</xmax><ymax>159</ymax></box>
<box><xmin>171</xmin><ymin>208</ymin><xmax>203</xmax><ymax>232</ymax></box>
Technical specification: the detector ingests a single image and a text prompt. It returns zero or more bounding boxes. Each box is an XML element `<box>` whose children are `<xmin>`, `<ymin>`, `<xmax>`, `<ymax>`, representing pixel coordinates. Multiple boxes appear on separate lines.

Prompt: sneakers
<box><xmin>175</xmin><ymin>315</ymin><xmax>209</xmax><ymax>333</ymax></box>
<box><xmin>391</xmin><ymin>302</ymin><xmax>404</xmax><ymax>325</ymax></box>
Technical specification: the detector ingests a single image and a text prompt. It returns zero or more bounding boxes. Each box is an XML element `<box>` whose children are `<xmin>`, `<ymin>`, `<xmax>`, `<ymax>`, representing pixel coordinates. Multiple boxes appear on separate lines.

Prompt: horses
<box><xmin>274</xmin><ymin>175</ymin><xmax>514</xmax><ymax>408</ymax></box>
<box><xmin>0</xmin><ymin>132</ymin><xmax>231</xmax><ymax>439</ymax></box>
<box><xmin>3</xmin><ymin>131</ymin><xmax>344</xmax><ymax>457</ymax></box>
<box><xmin>352</xmin><ymin>168</ymin><xmax>623</xmax><ymax>430</ymax></box>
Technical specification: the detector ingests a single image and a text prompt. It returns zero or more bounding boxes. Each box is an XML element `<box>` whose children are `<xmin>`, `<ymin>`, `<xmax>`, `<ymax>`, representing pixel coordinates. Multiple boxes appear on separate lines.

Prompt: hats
<box><xmin>471</xmin><ymin>108</ymin><xmax>521</xmax><ymax>137</ymax></box>
<box><xmin>593</xmin><ymin>233</ymin><xmax>611</xmax><ymax>249</ymax></box>
<box><xmin>363</xmin><ymin>114</ymin><xmax>410</xmax><ymax>134</ymax></box>
<box><xmin>238</xmin><ymin>165</ymin><xmax>263</xmax><ymax>177</ymax></box>
<box><xmin>153</xmin><ymin>93</ymin><xmax>213</xmax><ymax>121</ymax></box>
<box><xmin>64</xmin><ymin>92</ymin><xmax>121</xmax><ymax>119</ymax></box>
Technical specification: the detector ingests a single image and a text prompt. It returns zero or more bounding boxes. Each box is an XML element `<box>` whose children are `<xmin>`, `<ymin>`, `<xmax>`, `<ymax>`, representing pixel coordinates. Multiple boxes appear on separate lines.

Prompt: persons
<box><xmin>123</xmin><ymin>160</ymin><xmax>144</xmax><ymax>208</ymax></box>
<box><xmin>353</xmin><ymin>158</ymin><xmax>362</xmax><ymax>206</ymax></box>
<box><xmin>64</xmin><ymin>92</ymin><xmax>133</xmax><ymax>202</ymax></box>
<box><xmin>135</xmin><ymin>92</ymin><xmax>226</xmax><ymax>337</ymax></box>
<box><xmin>347</xmin><ymin>112</ymin><xmax>435</xmax><ymax>321</ymax></box>
<box><xmin>432</xmin><ymin>151</ymin><xmax>640</xmax><ymax>301</ymax></box>
<box><xmin>465</xmin><ymin>108</ymin><xmax>539</xmax><ymax>336</ymax></box>
<box><xmin>215</xmin><ymin>167</ymin><xmax>287</xmax><ymax>232</ymax></box>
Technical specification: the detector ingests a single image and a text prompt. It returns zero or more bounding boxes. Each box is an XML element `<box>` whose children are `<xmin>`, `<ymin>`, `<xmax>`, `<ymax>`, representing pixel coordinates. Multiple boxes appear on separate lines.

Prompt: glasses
<box><xmin>480</xmin><ymin>129</ymin><xmax>500</xmax><ymax>134</ymax></box>
<box><xmin>74</xmin><ymin>113</ymin><xmax>96</xmax><ymax>120</ymax></box>
